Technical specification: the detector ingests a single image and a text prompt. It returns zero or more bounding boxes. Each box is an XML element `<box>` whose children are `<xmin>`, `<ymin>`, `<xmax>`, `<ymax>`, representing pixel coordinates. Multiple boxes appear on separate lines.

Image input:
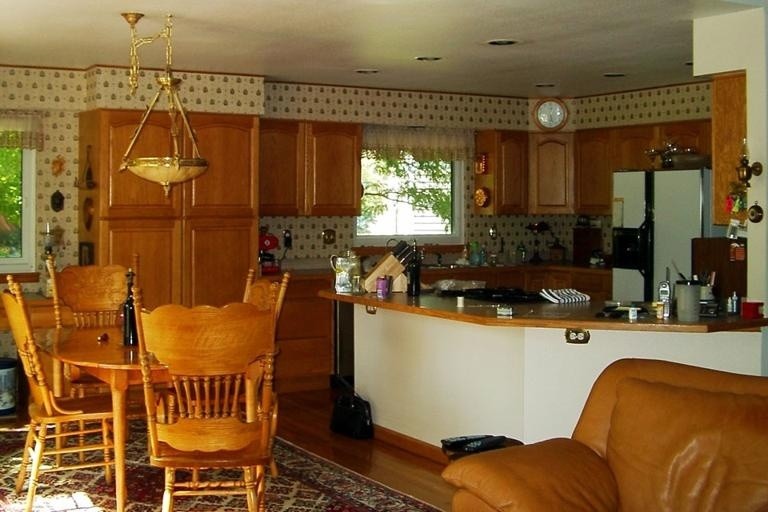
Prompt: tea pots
<box><xmin>330</xmin><ymin>250</ymin><xmax>361</xmax><ymax>294</ymax></box>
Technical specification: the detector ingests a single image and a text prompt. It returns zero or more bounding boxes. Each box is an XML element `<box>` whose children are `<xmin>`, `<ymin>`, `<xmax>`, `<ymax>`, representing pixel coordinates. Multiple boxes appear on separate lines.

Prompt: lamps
<box><xmin>118</xmin><ymin>13</ymin><xmax>208</xmax><ymax>200</ymax></box>
<box><xmin>735</xmin><ymin>138</ymin><xmax>762</xmax><ymax>189</ymax></box>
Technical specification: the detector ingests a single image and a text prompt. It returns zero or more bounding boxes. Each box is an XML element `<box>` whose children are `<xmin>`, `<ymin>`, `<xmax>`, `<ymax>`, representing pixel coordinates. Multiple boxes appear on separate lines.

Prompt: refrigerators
<box><xmin>610</xmin><ymin>168</ymin><xmax>729</xmax><ymax>304</ymax></box>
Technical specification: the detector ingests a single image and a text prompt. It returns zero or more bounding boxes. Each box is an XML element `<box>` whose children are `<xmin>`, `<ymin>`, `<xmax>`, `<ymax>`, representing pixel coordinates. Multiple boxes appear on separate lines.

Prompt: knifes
<box><xmin>392</xmin><ymin>240</ymin><xmax>411</xmax><ymax>267</ymax></box>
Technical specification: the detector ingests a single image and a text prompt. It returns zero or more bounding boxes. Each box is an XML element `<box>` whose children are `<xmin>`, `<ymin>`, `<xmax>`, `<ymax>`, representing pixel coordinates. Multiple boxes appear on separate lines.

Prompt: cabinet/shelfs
<box><xmin>575</xmin><ymin>120</ymin><xmax>711</xmax><ymax>216</ymax></box>
<box><xmin>274</xmin><ymin>276</ymin><xmax>334</xmax><ymax>391</ymax></box>
<box><xmin>711</xmin><ymin>73</ymin><xmax>749</xmax><ymax>227</ymax></box>
<box><xmin>474</xmin><ymin>129</ymin><xmax>528</xmax><ymax>216</ymax></box>
<box><xmin>261</xmin><ymin>120</ymin><xmax>362</xmax><ymax>217</ymax></box>
<box><xmin>77</xmin><ymin>108</ymin><xmax>263</xmax><ymax>307</ymax></box>
<box><xmin>529</xmin><ymin>131</ymin><xmax>612</xmax><ymax>216</ymax></box>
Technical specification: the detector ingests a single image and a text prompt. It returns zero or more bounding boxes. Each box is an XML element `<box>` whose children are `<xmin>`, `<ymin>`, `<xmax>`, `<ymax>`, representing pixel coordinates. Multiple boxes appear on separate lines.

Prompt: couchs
<box><xmin>441</xmin><ymin>357</ymin><xmax>767</xmax><ymax>511</ymax></box>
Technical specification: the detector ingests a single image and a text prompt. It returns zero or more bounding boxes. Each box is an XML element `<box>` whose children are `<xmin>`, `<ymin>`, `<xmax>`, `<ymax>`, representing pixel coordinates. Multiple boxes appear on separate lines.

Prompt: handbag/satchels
<box><xmin>329</xmin><ymin>395</ymin><xmax>373</xmax><ymax>440</ymax></box>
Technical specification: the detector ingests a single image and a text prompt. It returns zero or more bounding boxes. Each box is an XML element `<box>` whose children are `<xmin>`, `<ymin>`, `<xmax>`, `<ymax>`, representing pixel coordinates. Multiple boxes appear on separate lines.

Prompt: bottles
<box><xmin>352</xmin><ymin>274</ymin><xmax>366</xmax><ymax>296</ymax></box>
<box><xmin>517</xmin><ymin>241</ymin><xmax>526</xmax><ymax>264</ymax></box>
<box><xmin>376</xmin><ymin>276</ymin><xmax>389</xmax><ymax>297</ymax></box>
<box><xmin>463</xmin><ymin>241</ymin><xmax>497</xmax><ymax>266</ymax></box>
<box><xmin>41</xmin><ymin>222</ymin><xmax>57</xmax><ymax>299</ymax></box>
<box><xmin>409</xmin><ymin>238</ymin><xmax>421</xmax><ymax>297</ymax></box>
<box><xmin>629</xmin><ymin>308</ymin><xmax>638</xmax><ymax>321</ymax></box>
<box><xmin>124</xmin><ymin>269</ymin><xmax>138</xmax><ymax>349</ymax></box>
<box><xmin>728</xmin><ymin>291</ymin><xmax>739</xmax><ymax>314</ymax></box>
<box><xmin>656</xmin><ymin>299</ymin><xmax>670</xmax><ymax>319</ymax></box>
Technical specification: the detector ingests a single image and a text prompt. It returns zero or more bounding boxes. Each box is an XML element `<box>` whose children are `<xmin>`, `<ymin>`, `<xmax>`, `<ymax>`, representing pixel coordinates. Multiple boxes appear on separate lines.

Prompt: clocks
<box><xmin>532</xmin><ymin>97</ymin><xmax>568</xmax><ymax>131</ymax></box>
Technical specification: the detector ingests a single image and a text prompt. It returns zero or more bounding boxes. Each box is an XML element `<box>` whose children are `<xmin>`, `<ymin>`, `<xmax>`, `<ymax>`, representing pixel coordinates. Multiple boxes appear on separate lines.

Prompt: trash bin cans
<box><xmin>1</xmin><ymin>357</ymin><xmax>20</xmax><ymax>416</ymax></box>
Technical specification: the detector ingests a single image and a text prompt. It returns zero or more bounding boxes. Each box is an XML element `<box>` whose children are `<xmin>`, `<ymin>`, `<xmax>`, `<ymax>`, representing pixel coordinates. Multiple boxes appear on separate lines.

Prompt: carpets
<box><xmin>0</xmin><ymin>415</ymin><xmax>441</xmax><ymax>512</ymax></box>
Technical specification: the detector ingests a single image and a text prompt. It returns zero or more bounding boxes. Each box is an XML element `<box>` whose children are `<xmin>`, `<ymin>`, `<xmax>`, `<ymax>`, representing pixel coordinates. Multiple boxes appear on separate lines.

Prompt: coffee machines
<box><xmin>259</xmin><ymin>232</ymin><xmax>281</xmax><ymax>275</ymax></box>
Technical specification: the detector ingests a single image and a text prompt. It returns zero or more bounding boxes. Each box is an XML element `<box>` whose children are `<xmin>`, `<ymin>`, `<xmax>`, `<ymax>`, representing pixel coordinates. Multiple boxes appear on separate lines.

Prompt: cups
<box><xmin>742</xmin><ymin>301</ymin><xmax>764</xmax><ymax>319</ymax></box>
<box><xmin>676</xmin><ymin>280</ymin><xmax>702</xmax><ymax>324</ymax></box>
<box><xmin>456</xmin><ymin>296</ymin><xmax>466</xmax><ymax>308</ymax></box>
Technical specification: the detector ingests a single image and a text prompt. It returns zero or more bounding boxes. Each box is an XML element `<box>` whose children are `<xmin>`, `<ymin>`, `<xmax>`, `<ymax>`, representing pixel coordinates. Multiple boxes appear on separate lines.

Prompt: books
<box><xmin>538</xmin><ymin>287</ymin><xmax>591</xmax><ymax>304</ymax></box>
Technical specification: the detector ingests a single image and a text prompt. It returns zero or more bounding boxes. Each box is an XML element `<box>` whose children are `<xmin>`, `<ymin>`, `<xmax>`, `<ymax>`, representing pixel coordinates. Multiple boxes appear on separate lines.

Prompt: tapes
<box><xmin>748</xmin><ymin>205</ymin><xmax>763</xmax><ymax>223</ymax></box>
